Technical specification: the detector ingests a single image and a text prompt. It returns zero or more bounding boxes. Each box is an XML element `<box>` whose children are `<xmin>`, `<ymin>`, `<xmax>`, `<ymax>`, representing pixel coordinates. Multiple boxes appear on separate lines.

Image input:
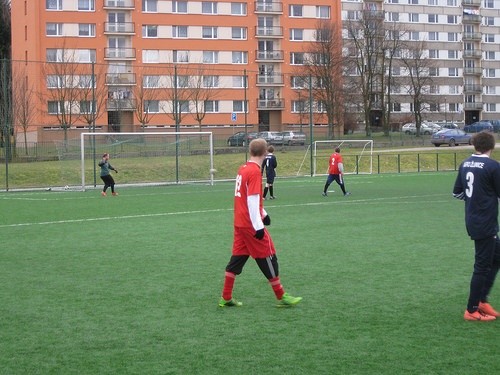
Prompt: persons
<box><xmin>218</xmin><ymin>138</ymin><xmax>303</xmax><ymax>307</ymax></box>
<box><xmin>98</xmin><ymin>153</ymin><xmax>118</xmax><ymax>196</ymax></box>
<box><xmin>321</xmin><ymin>148</ymin><xmax>352</xmax><ymax>197</ymax></box>
<box><xmin>452</xmin><ymin>130</ymin><xmax>500</xmax><ymax>322</ymax></box>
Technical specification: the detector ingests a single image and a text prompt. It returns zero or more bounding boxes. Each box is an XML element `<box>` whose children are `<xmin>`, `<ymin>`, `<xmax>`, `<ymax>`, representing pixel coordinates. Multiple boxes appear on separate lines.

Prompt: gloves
<box><xmin>262</xmin><ymin>215</ymin><xmax>270</xmax><ymax>225</ymax></box>
<box><xmin>114</xmin><ymin>170</ymin><xmax>118</xmax><ymax>173</ymax></box>
<box><xmin>253</xmin><ymin>228</ymin><xmax>264</xmax><ymax>240</ymax></box>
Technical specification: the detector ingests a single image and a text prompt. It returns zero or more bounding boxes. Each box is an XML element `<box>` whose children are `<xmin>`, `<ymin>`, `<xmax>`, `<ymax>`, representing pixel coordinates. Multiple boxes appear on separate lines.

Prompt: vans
<box><xmin>481</xmin><ymin>119</ymin><xmax>500</xmax><ymax>132</ymax></box>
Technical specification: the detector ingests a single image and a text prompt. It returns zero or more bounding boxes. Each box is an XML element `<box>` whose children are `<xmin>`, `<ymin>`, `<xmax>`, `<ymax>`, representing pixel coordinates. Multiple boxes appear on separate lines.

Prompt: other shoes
<box><xmin>344</xmin><ymin>192</ymin><xmax>352</xmax><ymax>196</ymax></box>
<box><xmin>112</xmin><ymin>192</ymin><xmax>118</xmax><ymax>196</ymax></box>
<box><xmin>262</xmin><ymin>197</ymin><xmax>276</xmax><ymax>200</ymax></box>
<box><xmin>322</xmin><ymin>193</ymin><xmax>327</xmax><ymax>197</ymax></box>
<box><xmin>101</xmin><ymin>191</ymin><xmax>106</xmax><ymax>196</ymax></box>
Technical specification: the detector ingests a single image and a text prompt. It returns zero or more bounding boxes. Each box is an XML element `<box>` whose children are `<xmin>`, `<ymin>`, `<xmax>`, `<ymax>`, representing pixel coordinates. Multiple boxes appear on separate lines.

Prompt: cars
<box><xmin>465</xmin><ymin>122</ymin><xmax>493</xmax><ymax>132</ymax></box>
<box><xmin>401</xmin><ymin>121</ymin><xmax>459</xmax><ymax>134</ymax></box>
<box><xmin>431</xmin><ymin>130</ymin><xmax>472</xmax><ymax>146</ymax></box>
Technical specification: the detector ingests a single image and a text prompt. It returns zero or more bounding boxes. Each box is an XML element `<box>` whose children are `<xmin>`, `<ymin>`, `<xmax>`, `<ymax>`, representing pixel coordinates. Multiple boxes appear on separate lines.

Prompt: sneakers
<box><xmin>219</xmin><ymin>296</ymin><xmax>242</xmax><ymax>307</ymax></box>
<box><xmin>276</xmin><ymin>294</ymin><xmax>303</xmax><ymax>308</ymax></box>
<box><xmin>464</xmin><ymin>302</ymin><xmax>500</xmax><ymax>321</ymax></box>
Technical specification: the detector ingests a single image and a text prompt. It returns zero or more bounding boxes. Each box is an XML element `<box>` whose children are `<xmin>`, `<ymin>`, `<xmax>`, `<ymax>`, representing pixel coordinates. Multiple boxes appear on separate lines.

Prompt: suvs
<box><xmin>282</xmin><ymin>131</ymin><xmax>306</xmax><ymax>146</ymax></box>
<box><xmin>228</xmin><ymin>131</ymin><xmax>258</xmax><ymax>147</ymax></box>
<box><xmin>258</xmin><ymin>132</ymin><xmax>283</xmax><ymax>146</ymax></box>
